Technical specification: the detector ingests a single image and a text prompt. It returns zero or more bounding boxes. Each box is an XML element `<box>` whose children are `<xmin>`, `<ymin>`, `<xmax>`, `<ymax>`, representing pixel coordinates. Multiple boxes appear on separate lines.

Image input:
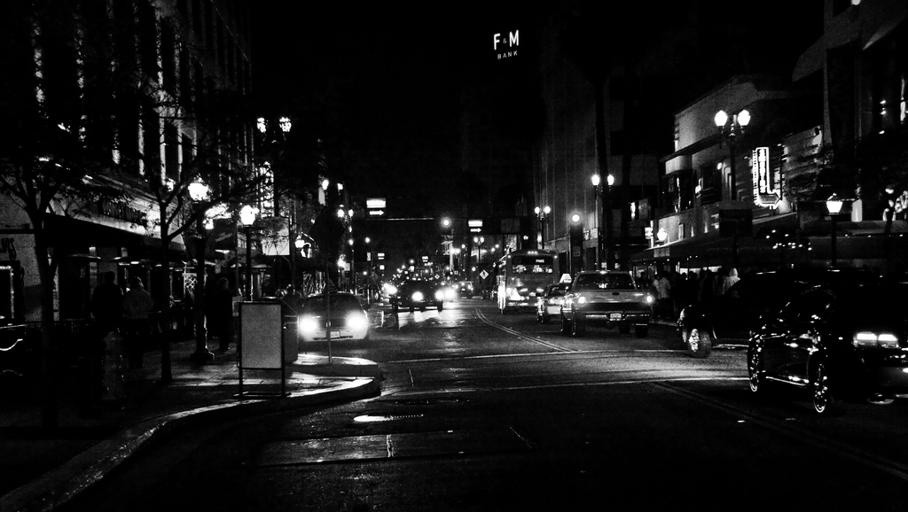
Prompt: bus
<box><xmin>494</xmin><ymin>248</ymin><xmax>555</xmax><ymax>314</ymax></box>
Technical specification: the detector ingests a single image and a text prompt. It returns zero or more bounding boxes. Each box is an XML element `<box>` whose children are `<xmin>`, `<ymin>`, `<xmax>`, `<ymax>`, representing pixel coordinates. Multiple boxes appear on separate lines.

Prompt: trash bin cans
<box><xmin>285</xmin><ymin>317</ymin><xmax>298</xmax><ymax>365</ymax></box>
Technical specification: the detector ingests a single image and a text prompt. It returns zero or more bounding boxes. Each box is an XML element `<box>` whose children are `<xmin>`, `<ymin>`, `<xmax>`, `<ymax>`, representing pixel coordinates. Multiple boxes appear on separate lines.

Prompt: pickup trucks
<box><xmin>558</xmin><ymin>269</ymin><xmax>653</xmax><ymax>338</ymax></box>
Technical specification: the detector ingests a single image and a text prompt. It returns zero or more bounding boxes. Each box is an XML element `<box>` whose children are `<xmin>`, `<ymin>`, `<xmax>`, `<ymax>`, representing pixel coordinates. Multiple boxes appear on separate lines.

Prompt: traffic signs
<box><xmin>465</xmin><ymin>219</ymin><xmax>484</xmax><ymax>233</ymax></box>
<box><xmin>366</xmin><ymin>194</ymin><xmax>388</xmax><ymax>217</ymax></box>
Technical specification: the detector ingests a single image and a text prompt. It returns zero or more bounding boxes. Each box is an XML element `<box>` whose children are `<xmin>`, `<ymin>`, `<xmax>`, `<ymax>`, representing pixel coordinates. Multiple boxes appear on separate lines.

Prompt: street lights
<box><xmin>473</xmin><ymin>235</ymin><xmax>485</xmax><ymax>285</ymax></box>
<box><xmin>826</xmin><ymin>191</ymin><xmax>845</xmax><ymax>269</ymax></box>
<box><xmin>188</xmin><ymin>181</ymin><xmax>216</xmax><ymax>363</ymax></box>
<box><xmin>256</xmin><ymin>115</ymin><xmax>293</xmax><ymax>213</ymax></box>
<box><xmin>238</xmin><ymin>202</ymin><xmax>257</xmax><ymax>302</ymax></box>
<box><xmin>714</xmin><ymin>106</ymin><xmax>752</xmax><ymax>201</ymax></box>
<box><xmin>591</xmin><ymin>173</ymin><xmax>615</xmax><ymax>266</ymax></box>
<box><xmin>533</xmin><ymin>206</ymin><xmax>552</xmax><ymax>249</ymax></box>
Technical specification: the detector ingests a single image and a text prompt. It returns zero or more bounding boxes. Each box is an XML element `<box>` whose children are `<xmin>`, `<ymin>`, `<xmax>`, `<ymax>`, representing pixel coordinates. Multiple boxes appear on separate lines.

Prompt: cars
<box><xmin>388</xmin><ymin>279</ymin><xmax>443</xmax><ymax>314</ymax></box>
<box><xmin>676</xmin><ymin>268</ymin><xmax>823</xmax><ymax>358</ymax></box>
<box><xmin>296</xmin><ymin>293</ymin><xmax>371</xmax><ymax>345</ymax></box>
<box><xmin>374</xmin><ymin>272</ymin><xmax>472</xmax><ymax>304</ymax></box>
<box><xmin>745</xmin><ymin>280</ymin><xmax>908</xmax><ymax>414</ymax></box>
<box><xmin>536</xmin><ymin>283</ymin><xmax>573</xmax><ymax>322</ymax></box>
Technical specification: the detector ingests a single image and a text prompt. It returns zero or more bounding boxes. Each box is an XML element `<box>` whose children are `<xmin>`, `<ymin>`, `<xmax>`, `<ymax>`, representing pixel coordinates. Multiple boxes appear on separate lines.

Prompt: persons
<box><xmin>634</xmin><ymin>263</ymin><xmax>729</xmax><ymax>324</ymax></box>
<box><xmin>89</xmin><ymin>270</ymin><xmax>120</xmax><ymax>365</ymax></box>
<box><xmin>205</xmin><ymin>274</ymin><xmax>234</xmax><ymax>354</ymax></box>
<box><xmin>116</xmin><ymin>274</ymin><xmax>157</xmax><ymax>366</ymax></box>
